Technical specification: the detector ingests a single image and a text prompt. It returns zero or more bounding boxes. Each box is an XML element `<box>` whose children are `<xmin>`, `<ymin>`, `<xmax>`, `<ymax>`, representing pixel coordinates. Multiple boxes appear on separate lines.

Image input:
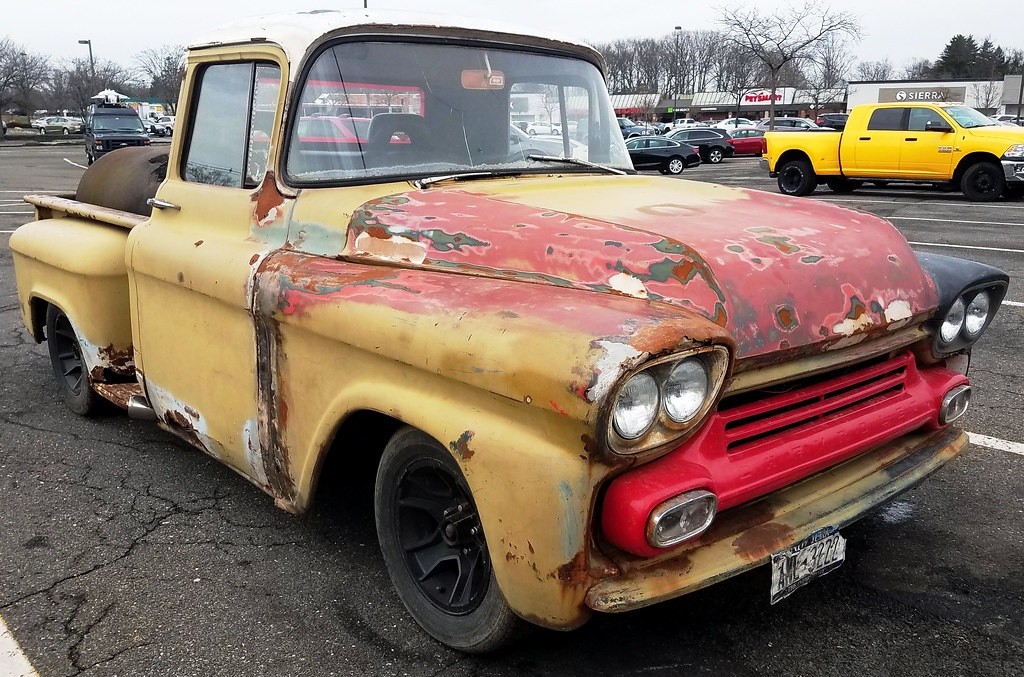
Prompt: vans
<box><xmin>577</xmin><ymin>118</ymin><xmax>656</xmax><ymax>146</ymax></box>
<box><xmin>816</xmin><ymin>112</ymin><xmax>849</xmax><ymax>129</ymax></box>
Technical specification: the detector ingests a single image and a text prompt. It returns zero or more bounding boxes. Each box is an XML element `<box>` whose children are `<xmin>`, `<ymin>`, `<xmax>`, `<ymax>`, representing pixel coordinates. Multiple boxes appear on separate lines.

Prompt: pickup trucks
<box><xmin>760</xmin><ymin>100</ymin><xmax>1024</xmax><ymax>203</ymax></box>
<box><xmin>7</xmin><ymin>9</ymin><xmax>1010</xmax><ymax>659</ymax></box>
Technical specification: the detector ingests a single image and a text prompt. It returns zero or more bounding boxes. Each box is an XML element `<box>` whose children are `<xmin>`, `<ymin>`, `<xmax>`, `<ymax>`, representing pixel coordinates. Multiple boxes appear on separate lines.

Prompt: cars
<box><xmin>636</xmin><ymin>114</ymin><xmax>759</xmax><ymax>135</ymax></box>
<box><xmin>508</xmin><ymin>121</ymin><xmax>585</xmax><ymax>160</ymax></box>
<box><xmin>567</xmin><ymin>120</ymin><xmax>579</xmax><ymax>133</ymax></box>
<box><xmin>30</xmin><ymin>116</ymin><xmax>49</xmax><ymax>128</ymax></box>
<box><xmin>38</xmin><ymin>116</ymin><xmax>85</xmax><ymax>135</ymax></box>
<box><xmin>252</xmin><ymin>114</ymin><xmax>411</xmax><ymax>144</ymax></box>
<box><xmin>526</xmin><ymin>119</ymin><xmax>562</xmax><ymax>136</ymax></box>
<box><xmin>154</xmin><ymin>116</ymin><xmax>177</xmax><ymax>137</ymax></box>
<box><xmin>141</xmin><ymin>118</ymin><xmax>167</xmax><ymax>138</ymax></box>
<box><xmin>512</xmin><ymin>120</ymin><xmax>528</xmax><ymax>133</ymax></box>
<box><xmin>725</xmin><ymin>129</ymin><xmax>768</xmax><ymax>156</ymax></box>
<box><xmin>624</xmin><ymin>135</ymin><xmax>701</xmax><ymax>176</ymax></box>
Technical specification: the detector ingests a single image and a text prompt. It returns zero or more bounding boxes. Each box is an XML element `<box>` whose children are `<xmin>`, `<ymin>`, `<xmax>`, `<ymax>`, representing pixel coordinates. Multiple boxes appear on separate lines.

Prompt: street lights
<box><xmin>20</xmin><ymin>52</ymin><xmax>33</xmax><ymax>126</ymax></box>
<box><xmin>78</xmin><ymin>37</ymin><xmax>99</xmax><ymax>97</ymax></box>
<box><xmin>672</xmin><ymin>25</ymin><xmax>682</xmax><ymax>130</ymax></box>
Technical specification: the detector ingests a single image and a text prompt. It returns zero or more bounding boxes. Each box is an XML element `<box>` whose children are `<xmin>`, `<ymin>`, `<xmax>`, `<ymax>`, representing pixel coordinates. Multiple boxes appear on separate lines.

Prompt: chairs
<box><xmin>362</xmin><ymin>111</ymin><xmax>440</xmax><ymax>170</ymax></box>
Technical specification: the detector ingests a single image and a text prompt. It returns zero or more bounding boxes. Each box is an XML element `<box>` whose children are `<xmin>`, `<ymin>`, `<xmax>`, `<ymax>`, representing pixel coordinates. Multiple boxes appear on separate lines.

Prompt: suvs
<box><xmin>662</xmin><ymin>126</ymin><xmax>736</xmax><ymax>163</ymax></box>
<box><xmin>83</xmin><ymin>88</ymin><xmax>151</xmax><ymax>166</ymax></box>
<box><xmin>756</xmin><ymin>117</ymin><xmax>835</xmax><ymax>133</ymax></box>
